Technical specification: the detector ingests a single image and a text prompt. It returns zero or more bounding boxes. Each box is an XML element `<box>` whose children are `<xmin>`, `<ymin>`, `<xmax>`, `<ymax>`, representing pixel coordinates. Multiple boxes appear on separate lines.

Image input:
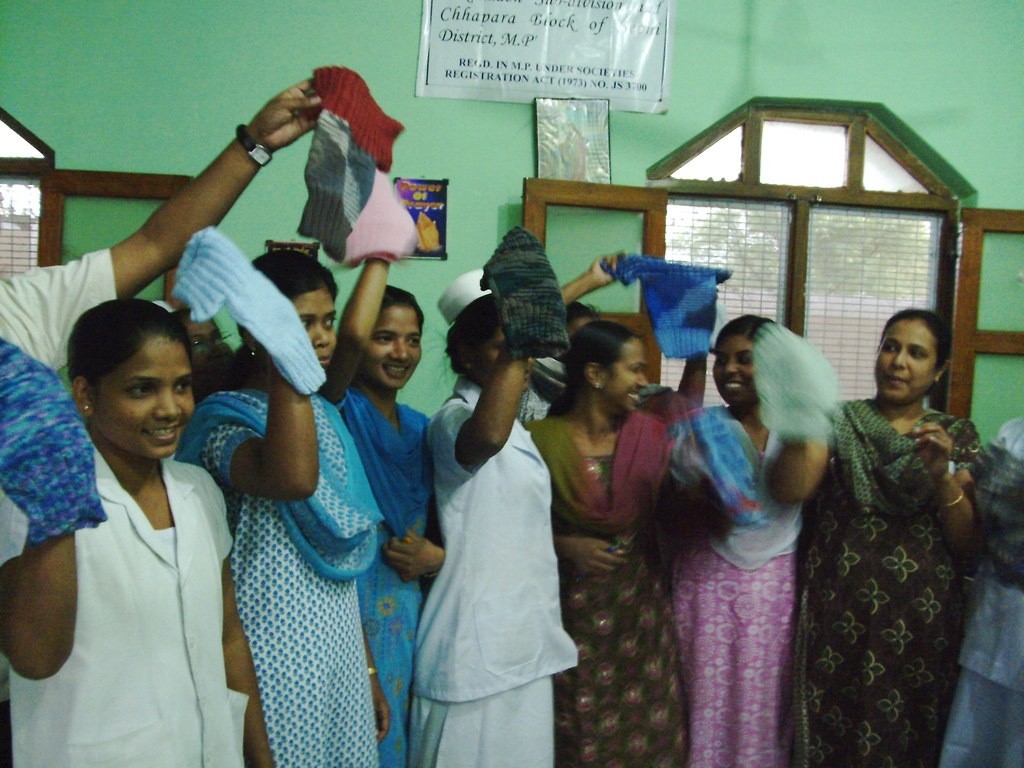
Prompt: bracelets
<box><xmin>943</xmin><ymin>491</ymin><xmax>963</xmax><ymax>507</ymax></box>
<box><xmin>368</xmin><ymin>667</ymin><xmax>378</xmax><ymax>676</ymax></box>
<box><xmin>424</xmin><ymin>573</ymin><xmax>437</xmax><ymax>577</ymax></box>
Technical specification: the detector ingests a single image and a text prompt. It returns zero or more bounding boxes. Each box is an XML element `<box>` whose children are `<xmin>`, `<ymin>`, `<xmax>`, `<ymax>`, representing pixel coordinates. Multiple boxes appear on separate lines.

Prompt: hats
<box><xmin>437</xmin><ymin>267</ymin><xmax>492</xmax><ymax>325</ymax></box>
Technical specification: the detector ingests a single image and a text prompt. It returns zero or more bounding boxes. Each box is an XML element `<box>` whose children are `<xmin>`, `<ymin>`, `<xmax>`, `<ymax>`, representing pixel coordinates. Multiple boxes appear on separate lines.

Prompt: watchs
<box><xmin>237</xmin><ymin>125</ymin><xmax>272</xmax><ymax>167</ymax></box>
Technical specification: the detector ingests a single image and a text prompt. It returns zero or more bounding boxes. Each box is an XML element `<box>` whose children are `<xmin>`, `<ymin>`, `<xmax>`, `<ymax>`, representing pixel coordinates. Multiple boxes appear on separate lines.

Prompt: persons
<box><xmin>524</xmin><ymin>321</ymin><xmax>690</xmax><ymax>768</ymax></box>
<box><xmin>0</xmin><ymin>298</ymin><xmax>277</xmax><ymax>768</ymax></box>
<box><xmin>317</xmin><ymin>257</ymin><xmax>446</xmax><ymax>768</ymax></box>
<box><xmin>0</xmin><ymin>77</ymin><xmax>324</xmax><ymax>372</ymax></box>
<box><xmin>170</xmin><ymin>307</ymin><xmax>228</xmax><ymax>404</ymax></box>
<box><xmin>175</xmin><ymin>251</ymin><xmax>390</xmax><ymax>768</ymax></box>
<box><xmin>766</xmin><ymin>309</ymin><xmax>986</xmax><ymax>768</ymax></box>
<box><xmin>939</xmin><ymin>418</ymin><xmax>1024</xmax><ymax>768</ymax></box>
<box><xmin>666</xmin><ymin>315</ymin><xmax>803</xmax><ymax>768</ymax></box>
<box><xmin>519</xmin><ymin>252</ymin><xmax>707</xmax><ymax>422</ymax></box>
<box><xmin>405</xmin><ymin>294</ymin><xmax>579</xmax><ymax>767</ymax></box>
